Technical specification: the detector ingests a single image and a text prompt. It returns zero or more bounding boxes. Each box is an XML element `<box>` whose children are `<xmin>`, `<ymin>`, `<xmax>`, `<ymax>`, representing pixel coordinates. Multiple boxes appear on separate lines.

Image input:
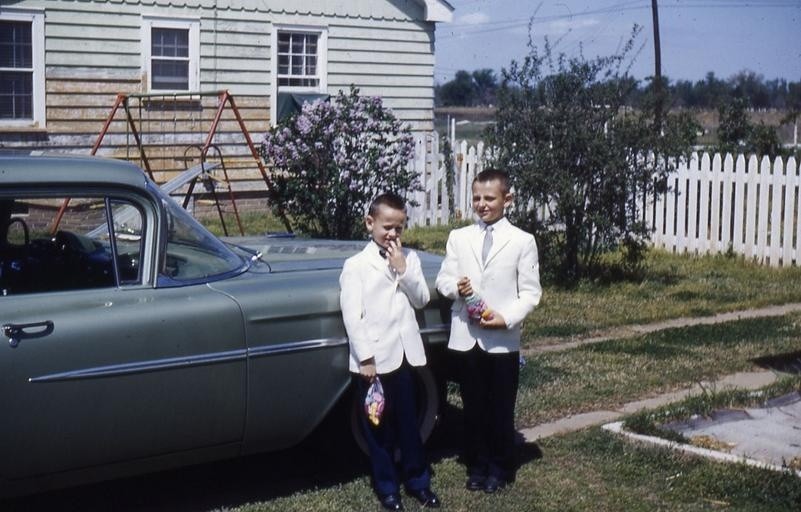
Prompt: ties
<box><xmin>482</xmin><ymin>225</ymin><xmax>493</xmax><ymax>264</ymax></box>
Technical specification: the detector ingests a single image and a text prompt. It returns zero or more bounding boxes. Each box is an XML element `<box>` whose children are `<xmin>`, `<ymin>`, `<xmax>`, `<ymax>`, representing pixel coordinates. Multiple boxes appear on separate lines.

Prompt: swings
<box><xmin>144</xmin><ymin>94</ymin><xmax>202</xmax><ymax>218</ymax></box>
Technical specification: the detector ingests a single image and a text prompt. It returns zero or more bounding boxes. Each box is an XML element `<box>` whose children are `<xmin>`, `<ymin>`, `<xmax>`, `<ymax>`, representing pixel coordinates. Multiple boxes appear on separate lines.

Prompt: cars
<box><xmin>2</xmin><ymin>156</ymin><xmax>452</xmax><ymax>501</ymax></box>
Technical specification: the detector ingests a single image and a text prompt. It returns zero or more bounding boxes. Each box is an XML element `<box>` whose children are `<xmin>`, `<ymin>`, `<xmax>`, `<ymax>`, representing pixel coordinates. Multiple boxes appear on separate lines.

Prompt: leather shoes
<box><xmin>380</xmin><ymin>493</ymin><xmax>402</xmax><ymax>511</ymax></box>
<box><xmin>412</xmin><ymin>488</ymin><xmax>439</xmax><ymax>508</ymax></box>
<box><xmin>467</xmin><ymin>473</ymin><xmax>501</xmax><ymax>493</ymax></box>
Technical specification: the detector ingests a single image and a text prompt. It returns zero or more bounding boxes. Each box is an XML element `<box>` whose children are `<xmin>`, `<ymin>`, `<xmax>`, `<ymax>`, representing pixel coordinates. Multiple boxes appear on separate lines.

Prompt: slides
<box><xmin>84</xmin><ymin>161</ymin><xmax>223</xmax><ymax>241</ymax></box>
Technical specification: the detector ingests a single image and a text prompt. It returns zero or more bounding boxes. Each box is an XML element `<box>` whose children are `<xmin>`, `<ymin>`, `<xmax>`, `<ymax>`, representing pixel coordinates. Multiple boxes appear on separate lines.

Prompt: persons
<box><xmin>435</xmin><ymin>167</ymin><xmax>543</xmax><ymax>493</ymax></box>
<box><xmin>339</xmin><ymin>193</ymin><xmax>440</xmax><ymax>512</ymax></box>
